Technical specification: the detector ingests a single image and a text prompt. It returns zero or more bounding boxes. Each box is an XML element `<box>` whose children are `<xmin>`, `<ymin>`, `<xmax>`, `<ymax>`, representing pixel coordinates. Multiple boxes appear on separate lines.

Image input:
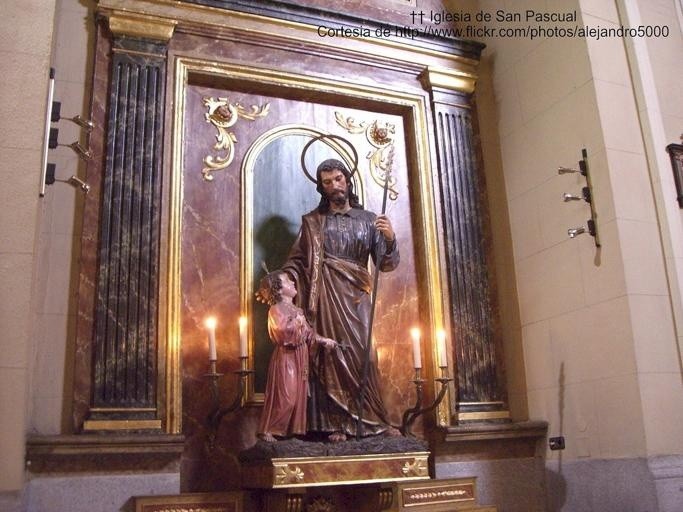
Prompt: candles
<box><xmin>207</xmin><ymin>317</ymin><xmax>216</xmax><ymax>360</ymax></box>
<box><xmin>240</xmin><ymin>318</ymin><xmax>248</xmax><ymax>357</ymax></box>
<box><xmin>438</xmin><ymin>332</ymin><xmax>447</xmax><ymax>366</ymax></box>
<box><xmin>412</xmin><ymin>329</ymin><xmax>422</xmax><ymax>368</ymax></box>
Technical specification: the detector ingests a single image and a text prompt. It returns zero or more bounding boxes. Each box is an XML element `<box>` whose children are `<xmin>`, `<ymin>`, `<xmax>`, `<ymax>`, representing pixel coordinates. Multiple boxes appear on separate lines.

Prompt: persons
<box><xmin>259</xmin><ymin>268</ymin><xmax>335</xmax><ymax>443</ymax></box>
<box><xmin>254</xmin><ymin>159</ymin><xmax>400</xmax><ymax>443</ymax></box>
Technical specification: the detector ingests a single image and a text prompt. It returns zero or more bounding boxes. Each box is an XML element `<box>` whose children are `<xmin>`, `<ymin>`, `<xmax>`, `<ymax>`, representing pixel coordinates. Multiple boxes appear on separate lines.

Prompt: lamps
<box><xmin>559</xmin><ymin>149</ymin><xmax>600</xmax><ymax>248</ymax></box>
<box><xmin>40</xmin><ymin>67</ymin><xmax>94</xmax><ymax>198</ymax></box>
